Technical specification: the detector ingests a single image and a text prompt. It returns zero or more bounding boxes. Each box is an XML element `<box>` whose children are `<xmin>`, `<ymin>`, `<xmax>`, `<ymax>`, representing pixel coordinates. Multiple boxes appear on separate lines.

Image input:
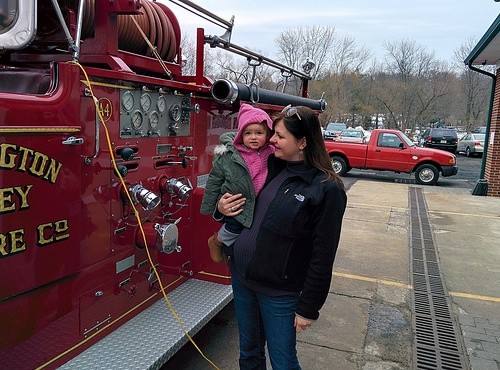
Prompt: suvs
<box><xmin>420</xmin><ymin>127</ymin><xmax>459</xmax><ymax>153</ymax></box>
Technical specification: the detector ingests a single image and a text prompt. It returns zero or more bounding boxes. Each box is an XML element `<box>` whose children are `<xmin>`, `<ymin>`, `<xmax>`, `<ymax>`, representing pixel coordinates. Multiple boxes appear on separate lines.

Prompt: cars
<box><xmin>321</xmin><ymin>122</ymin><xmax>403</xmax><ymax>147</ymax></box>
<box><xmin>456</xmin><ymin>132</ymin><xmax>485</xmax><ymax>158</ymax></box>
<box><xmin>405</xmin><ymin>126</ymin><xmax>487</xmax><ymax>148</ymax></box>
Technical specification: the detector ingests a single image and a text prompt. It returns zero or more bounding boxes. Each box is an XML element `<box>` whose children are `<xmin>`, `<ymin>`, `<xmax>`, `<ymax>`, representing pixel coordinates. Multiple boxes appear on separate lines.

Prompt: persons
<box><xmin>200</xmin><ymin>103</ymin><xmax>275</xmax><ymax>263</ymax></box>
<box><xmin>212</xmin><ymin>106</ymin><xmax>347</xmax><ymax>370</ymax></box>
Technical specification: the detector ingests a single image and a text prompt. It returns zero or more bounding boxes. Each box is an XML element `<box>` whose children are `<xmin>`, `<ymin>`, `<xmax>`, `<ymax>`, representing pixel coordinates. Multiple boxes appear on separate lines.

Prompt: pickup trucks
<box><xmin>323</xmin><ymin>128</ymin><xmax>459</xmax><ymax>186</ymax></box>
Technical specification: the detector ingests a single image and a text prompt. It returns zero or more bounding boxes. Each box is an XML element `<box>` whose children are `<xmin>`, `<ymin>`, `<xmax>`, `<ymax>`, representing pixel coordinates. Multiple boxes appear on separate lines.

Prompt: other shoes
<box><xmin>208</xmin><ymin>233</ymin><xmax>223</xmax><ymax>263</ymax></box>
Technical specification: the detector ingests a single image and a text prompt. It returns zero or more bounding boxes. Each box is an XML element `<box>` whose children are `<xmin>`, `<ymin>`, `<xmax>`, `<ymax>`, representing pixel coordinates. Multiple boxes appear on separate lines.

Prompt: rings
<box><xmin>306</xmin><ymin>326</ymin><xmax>308</xmax><ymax>329</ymax></box>
<box><xmin>230</xmin><ymin>208</ymin><xmax>232</xmax><ymax>212</ymax></box>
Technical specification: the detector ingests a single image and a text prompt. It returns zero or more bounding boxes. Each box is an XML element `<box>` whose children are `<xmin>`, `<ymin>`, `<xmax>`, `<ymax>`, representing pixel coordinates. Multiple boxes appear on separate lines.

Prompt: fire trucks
<box><xmin>0</xmin><ymin>0</ymin><xmax>327</xmax><ymax>370</ymax></box>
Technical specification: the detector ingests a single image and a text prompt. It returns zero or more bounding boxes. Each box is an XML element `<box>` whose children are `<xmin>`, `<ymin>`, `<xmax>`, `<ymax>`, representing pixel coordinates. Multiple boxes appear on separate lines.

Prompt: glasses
<box><xmin>280</xmin><ymin>104</ymin><xmax>302</xmax><ymax>121</ymax></box>
